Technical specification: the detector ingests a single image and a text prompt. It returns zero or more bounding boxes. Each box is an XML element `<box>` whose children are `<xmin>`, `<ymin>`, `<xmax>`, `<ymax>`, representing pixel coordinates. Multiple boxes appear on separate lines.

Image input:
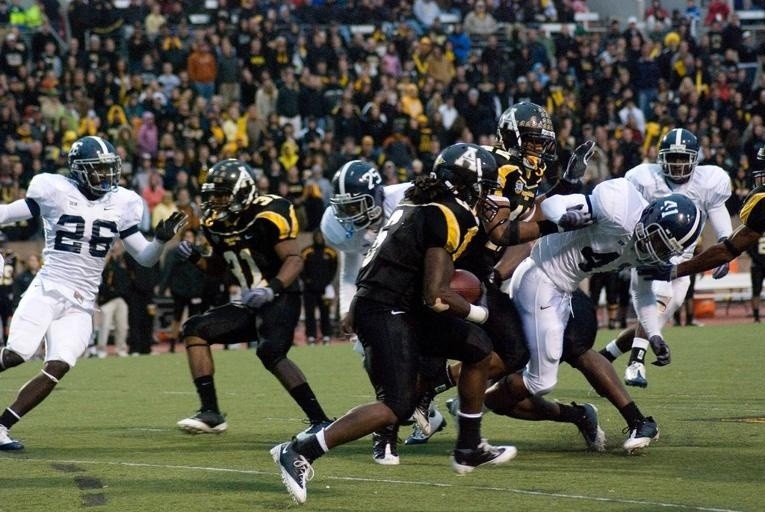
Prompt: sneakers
<box><xmin>412</xmin><ymin>392</ymin><xmax>433</xmax><ymax>435</ymax></box>
<box><xmin>624</xmin><ymin>362</ymin><xmax>647</xmax><ymax>388</ymax></box>
<box><xmin>296</xmin><ymin>417</ymin><xmax>337</xmax><ymax>440</ymax></box>
<box><xmin>177</xmin><ymin>411</ymin><xmax>228</xmax><ymax>433</ymax></box>
<box><xmin>405</xmin><ymin>409</ymin><xmax>447</xmax><ymax>444</ymax></box>
<box><xmin>445</xmin><ymin>399</ymin><xmax>458</xmax><ymax>423</ymax></box>
<box><xmin>0</xmin><ymin>424</ymin><xmax>23</xmax><ymax>450</ymax></box>
<box><xmin>451</xmin><ymin>438</ymin><xmax>516</xmax><ymax>472</ymax></box>
<box><xmin>571</xmin><ymin>400</ymin><xmax>605</xmax><ymax>453</ymax></box>
<box><xmin>269</xmin><ymin>436</ymin><xmax>315</xmax><ymax>504</ymax></box>
<box><xmin>622</xmin><ymin>416</ymin><xmax>660</xmax><ymax>450</ymax></box>
<box><xmin>373</xmin><ymin>424</ymin><xmax>402</xmax><ymax>465</ymax></box>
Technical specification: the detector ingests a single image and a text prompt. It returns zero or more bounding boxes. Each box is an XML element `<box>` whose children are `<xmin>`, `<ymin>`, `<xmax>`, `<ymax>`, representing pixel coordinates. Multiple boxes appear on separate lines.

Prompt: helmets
<box><xmin>329</xmin><ymin>160</ymin><xmax>384</xmax><ymax>233</ymax></box>
<box><xmin>752</xmin><ymin>147</ymin><xmax>765</xmax><ymax>187</ymax></box>
<box><xmin>200</xmin><ymin>158</ymin><xmax>256</xmax><ymax>227</ymax></box>
<box><xmin>68</xmin><ymin>136</ymin><xmax>122</xmax><ymax>197</ymax></box>
<box><xmin>658</xmin><ymin>127</ymin><xmax>699</xmax><ymax>183</ymax></box>
<box><xmin>634</xmin><ymin>194</ymin><xmax>702</xmax><ymax>266</ymax></box>
<box><xmin>429</xmin><ymin>143</ymin><xmax>499</xmax><ymax>223</ymax></box>
<box><xmin>496</xmin><ymin>102</ymin><xmax>558</xmax><ymax>172</ymax></box>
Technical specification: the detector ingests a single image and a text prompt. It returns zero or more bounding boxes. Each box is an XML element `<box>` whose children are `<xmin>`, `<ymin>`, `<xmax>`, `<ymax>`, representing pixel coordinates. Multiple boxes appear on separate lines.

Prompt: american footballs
<box><xmin>446</xmin><ymin>270</ymin><xmax>483</xmax><ymax>304</ymax></box>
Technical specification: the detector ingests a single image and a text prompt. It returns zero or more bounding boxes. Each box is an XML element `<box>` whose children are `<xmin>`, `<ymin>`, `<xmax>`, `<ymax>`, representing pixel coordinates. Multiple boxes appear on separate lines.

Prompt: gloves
<box><xmin>154</xmin><ymin>211</ymin><xmax>189</xmax><ymax>244</ymax></box>
<box><xmin>563</xmin><ymin>139</ymin><xmax>597</xmax><ymax>184</ymax></box>
<box><xmin>478</xmin><ymin>282</ymin><xmax>489</xmax><ymax>324</ymax></box>
<box><xmin>649</xmin><ymin>336</ymin><xmax>671</xmax><ymax>366</ymax></box>
<box><xmin>241</xmin><ymin>288</ymin><xmax>274</xmax><ymax>308</ymax></box>
<box><xmin>176</xmin><ymin>241</ymin><xmax>193</xmax><ymax>259</ymax></box>
<box><xmin>560</xmin><ymin>205</ymin><xmax>594</xmax><ymax>231</ymax></box>
<box><xmin>637</xmin><ymin>262</ymin><xmax>676</xmax><ymax>282</ymax></box>
<box><xmin>713</xmin><ymin>263</ymin><xmax>730</xmax><ymax>279</ymax></box>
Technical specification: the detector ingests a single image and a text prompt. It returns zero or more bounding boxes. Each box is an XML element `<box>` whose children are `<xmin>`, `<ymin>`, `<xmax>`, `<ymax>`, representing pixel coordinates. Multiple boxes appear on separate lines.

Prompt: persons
<box><xmin>1</xmin><ymin>2</ymin><xmax>764</xmax><ymax>503</ymax></box>
